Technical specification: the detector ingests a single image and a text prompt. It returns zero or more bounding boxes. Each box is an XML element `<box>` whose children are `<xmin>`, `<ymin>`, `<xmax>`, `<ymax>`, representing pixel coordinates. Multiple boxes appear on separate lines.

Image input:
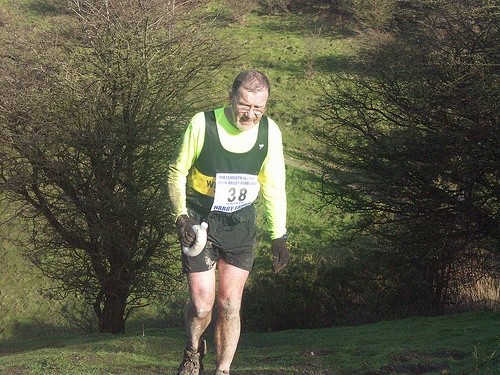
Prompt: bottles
<box><xmin>182</xmin><ymin>222</ymin><xmax>208</xmax><ymax>257</ymax></box>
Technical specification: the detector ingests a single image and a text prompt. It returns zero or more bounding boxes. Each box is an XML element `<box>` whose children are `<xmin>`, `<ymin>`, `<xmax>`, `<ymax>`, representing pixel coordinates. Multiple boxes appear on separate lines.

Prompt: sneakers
<box><xmin>176</xmin><ymin>338</ymin><xmax>207</xmax><ymax>375</ymax></box>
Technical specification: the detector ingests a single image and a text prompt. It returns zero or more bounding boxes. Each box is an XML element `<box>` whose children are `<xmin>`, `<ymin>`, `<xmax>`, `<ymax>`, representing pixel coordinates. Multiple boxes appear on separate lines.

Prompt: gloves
<box><xmin>175</xmin><ymin>214</ymin><xmax>199</xmax><ymax>247</ymax></box>
<box><xmin>271</xmin><ymin>237</ymin><xmax>289</xmax><ymax>272</ymax></box>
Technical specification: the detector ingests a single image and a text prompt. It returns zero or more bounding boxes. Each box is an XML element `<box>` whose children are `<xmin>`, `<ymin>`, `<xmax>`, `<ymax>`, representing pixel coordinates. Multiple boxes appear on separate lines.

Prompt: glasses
<box><xmin>233</xmin><ymin>95</ymin><xmax>266</xmax><ymax>114</ymax></box>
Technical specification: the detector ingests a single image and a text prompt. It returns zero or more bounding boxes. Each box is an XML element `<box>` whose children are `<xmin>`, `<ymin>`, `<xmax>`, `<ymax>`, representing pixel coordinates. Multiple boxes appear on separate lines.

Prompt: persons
<box><xmin>168</xmin><ymin>70</ymin><xmax>288</xmax><ymax>375</ymax></box>
